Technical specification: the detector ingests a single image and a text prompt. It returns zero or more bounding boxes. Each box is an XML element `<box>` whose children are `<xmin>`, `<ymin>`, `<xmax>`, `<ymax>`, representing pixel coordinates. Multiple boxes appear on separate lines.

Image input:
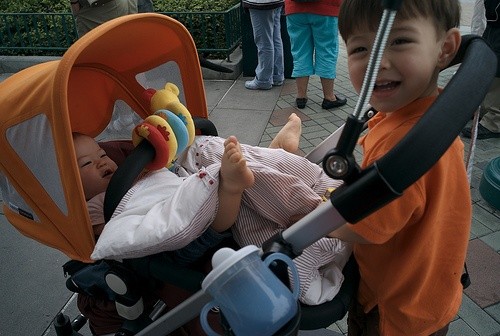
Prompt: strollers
<box><xmin>0</xmin><ymin>13</ymin><xmax>500</xmax><ymax>336</ymax></box>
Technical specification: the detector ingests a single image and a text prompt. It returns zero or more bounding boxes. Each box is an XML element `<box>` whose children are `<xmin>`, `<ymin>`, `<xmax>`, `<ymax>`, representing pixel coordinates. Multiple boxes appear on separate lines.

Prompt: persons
<box><xmin>290</xmin><ymin>0</ymin><xmax>474</xmax><ymax>336</ymax></box>
<box><xmin>68</xmin><ymin>0</ymin><xmax>500</xmax><ymax>141</ymax></box>
<box><xmin>71</xmin><ymin>112</ymin><xmax>304</xmax><ymax>238</ymax></box>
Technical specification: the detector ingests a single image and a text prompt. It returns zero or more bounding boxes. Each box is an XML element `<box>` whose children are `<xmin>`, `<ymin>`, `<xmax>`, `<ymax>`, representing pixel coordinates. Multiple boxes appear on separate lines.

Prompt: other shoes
<box><xmin>461</xmin><ymin>122</ymin><xmax>500</xmax><ymax>139</ymax></box>
<box><xmin>322</xmin><ymin>94</ymin><xmax>347</xmax><ymax>109</ymax></box>
<box><xmin>272</xmin><ymin>79</ymin><xmax>283</xmax><ymax>85</ymax></box>
<box><xmin>296</xmin><ymin>97</ymin><xmax>307</xmax><ymax>109</ymax></box>
<box><xmin>245</xmin><ymin>79</ymin><xmax>273</xmax><ymax>90</ymax></box>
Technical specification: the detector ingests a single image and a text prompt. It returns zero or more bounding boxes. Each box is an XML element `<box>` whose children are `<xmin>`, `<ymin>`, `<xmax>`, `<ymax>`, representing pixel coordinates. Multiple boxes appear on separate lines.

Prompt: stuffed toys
<box><xmin>133</xmin><ymin>80</ymin><xmax>196</xmax><ymax>166</ymax></box>
